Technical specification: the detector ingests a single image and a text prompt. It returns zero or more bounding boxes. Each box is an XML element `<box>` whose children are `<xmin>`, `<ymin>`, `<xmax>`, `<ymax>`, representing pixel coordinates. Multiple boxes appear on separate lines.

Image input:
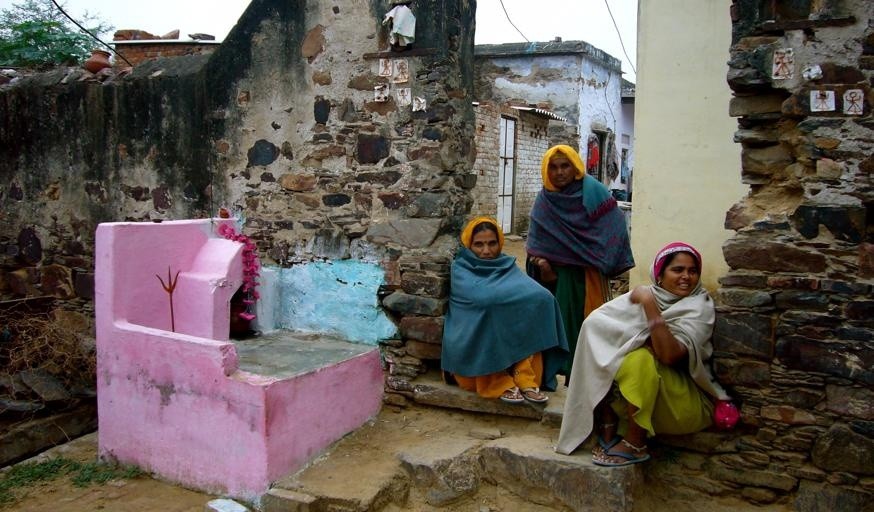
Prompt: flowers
<box><xmin>212</xmin><ymin>222</ymin><xmax>262</xmax><ymax>321</ymax></box>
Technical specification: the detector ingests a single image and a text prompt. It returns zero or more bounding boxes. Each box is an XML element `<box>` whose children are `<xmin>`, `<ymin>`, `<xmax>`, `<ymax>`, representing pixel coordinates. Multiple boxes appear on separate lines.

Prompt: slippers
<box><xmin>591</xmin><ymin>433</ymin><xmax>622</xmax><ymax>459</ymax></box>
<box><xmin>591</xmin><ymin>438</ymin><xmax>650</xmax><ymax>467</ymax></box>
<box><xmin>522</xmin><ymin>387</ymin><xmax>549</xmax><ymax>403</ymax></box>
<box><xmin>500</xmin><ymin>386</ymin><xmax>525</xmax><ymax>403</ymax></box>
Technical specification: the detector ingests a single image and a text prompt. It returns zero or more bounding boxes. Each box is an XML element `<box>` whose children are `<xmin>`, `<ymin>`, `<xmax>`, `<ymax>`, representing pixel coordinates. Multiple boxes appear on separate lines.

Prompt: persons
<box><xmin>523</xmin><ymin>144</ymin><xmax>637</xmax><ymax>388</ymax></box>
<box><xmin>438</xmin><ymin>217</ymin><xmax>572</xmax><ymax>406</ymax></box>
<box><xmin>552</xmin><ymin>240</ymin><xmax>742</xmax><ymax>468</ymax></box>
